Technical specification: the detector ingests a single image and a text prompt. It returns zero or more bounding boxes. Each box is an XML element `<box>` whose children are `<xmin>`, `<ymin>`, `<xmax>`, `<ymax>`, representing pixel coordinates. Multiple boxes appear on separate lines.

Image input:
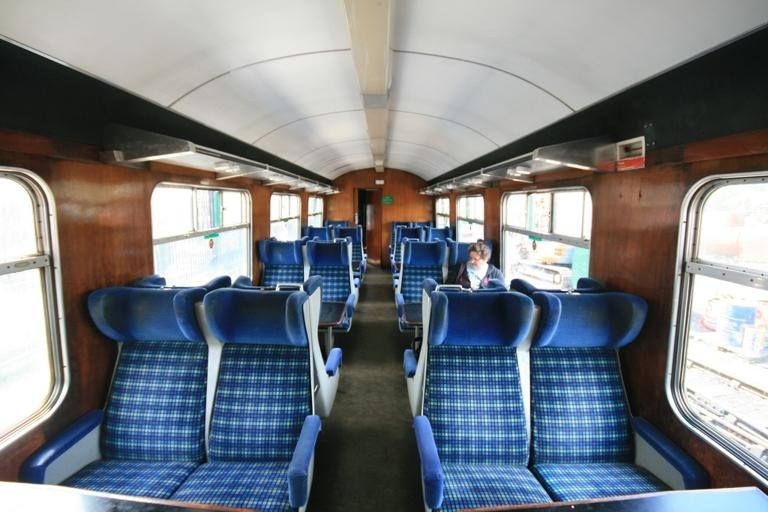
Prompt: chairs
<box><xmin>125</xmin><ymin>274</ymin><xmax>342</xmax><ymax>418</ymax></box>
<box><xmin>17</xmin><ymin>282</ymin><xmax>322</xmax><ymax>511</ymax></box>
<box><xmin>255</xmin><ymin>221</ymin><xmax>368</xmax><ymax>348</ymax></box>
<box><xmin>403</xmin><ymin>291</ymin><xmax>713</xmax><ymax>512</ymax></box>
<box><xmin>402</xmin><ymin>276</ymin><xmax>611</xmax><ymax>418</ymax></box>
<box><xmin>388</xmin><ymin>221</ymin><xmax>495</xmax><ymax>333</ymax></box>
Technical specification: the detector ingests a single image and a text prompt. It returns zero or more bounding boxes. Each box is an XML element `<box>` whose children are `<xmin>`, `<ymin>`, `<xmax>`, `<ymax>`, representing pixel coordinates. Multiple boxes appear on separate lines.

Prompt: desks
<box><xmin>318</xmin><ymin>301</ymin><xmax>344</xmax><ymax>356</ymax></box>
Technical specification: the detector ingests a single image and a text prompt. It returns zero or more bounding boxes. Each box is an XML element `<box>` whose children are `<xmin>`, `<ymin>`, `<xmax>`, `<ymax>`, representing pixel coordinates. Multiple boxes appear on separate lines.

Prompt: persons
<box><xmin>444</xmin><ymin>243</ymin><xmax>505</xmax><ymax>290</ymax></box>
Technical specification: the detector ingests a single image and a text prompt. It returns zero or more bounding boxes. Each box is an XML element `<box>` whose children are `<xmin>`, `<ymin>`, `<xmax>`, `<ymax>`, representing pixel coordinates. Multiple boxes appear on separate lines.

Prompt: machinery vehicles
<box><xmin>510</xmin><ymin>206</ymin><xmax>587</xmax><ymax>289</ymax></box>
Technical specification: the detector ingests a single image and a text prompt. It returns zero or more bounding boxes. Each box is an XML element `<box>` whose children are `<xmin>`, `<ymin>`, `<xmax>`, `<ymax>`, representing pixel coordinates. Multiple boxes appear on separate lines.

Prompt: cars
<box><xmin>690</xmin><ymin>270</ymin><xmax>767</xmax><ymax>363</ymax></box>
